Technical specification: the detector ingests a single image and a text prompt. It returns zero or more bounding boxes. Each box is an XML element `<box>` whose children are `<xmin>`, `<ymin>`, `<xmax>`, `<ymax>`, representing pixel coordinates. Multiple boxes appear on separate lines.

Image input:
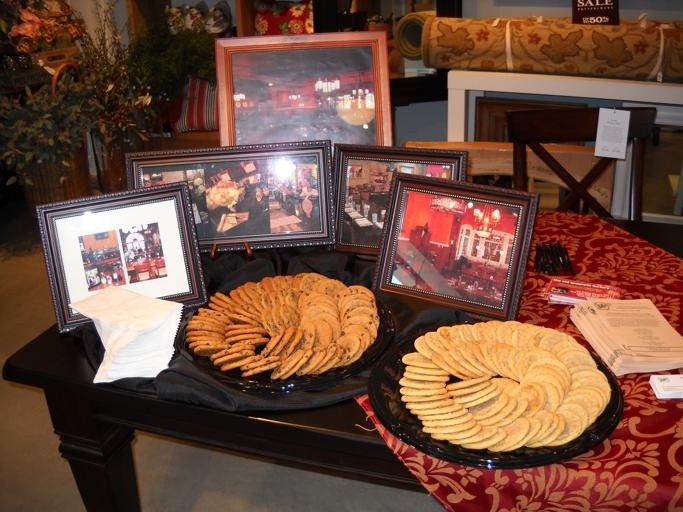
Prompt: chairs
<box><xmin>507</xmin><ymin>108</ymin><xmax>656</xmax><ymax>238</ymax></box>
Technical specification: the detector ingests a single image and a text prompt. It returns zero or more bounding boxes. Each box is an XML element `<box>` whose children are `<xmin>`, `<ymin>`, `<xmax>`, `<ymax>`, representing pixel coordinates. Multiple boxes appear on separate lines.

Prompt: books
<box><xmin>570</xmin><ymin>299</ymin><xmax>683</xmax><ymax>377</ymax></box>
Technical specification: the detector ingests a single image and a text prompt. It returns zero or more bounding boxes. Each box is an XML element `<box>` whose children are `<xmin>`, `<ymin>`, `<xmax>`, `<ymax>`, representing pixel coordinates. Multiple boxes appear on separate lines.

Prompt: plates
<box><xmin>175</xmin><ymin>298</ymin><xmax>396</xmax><ymax>399</ymax></box>
<box><xmin>371</xmin><ymin>327</ymin><xmax>621</xmax><ymax>468</ymax></box>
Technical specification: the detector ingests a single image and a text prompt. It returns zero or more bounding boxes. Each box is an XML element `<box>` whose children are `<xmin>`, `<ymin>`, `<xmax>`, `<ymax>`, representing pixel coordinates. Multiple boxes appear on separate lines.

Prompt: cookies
<box><xmin>398</xmin><ymin>320</ymin><xmax>611</xmax><ymax>453</ymax></box>
<box><xmin>186</xmin><ymin>272</ymin><xmax>379</xmax><ymax>380</ymax></box>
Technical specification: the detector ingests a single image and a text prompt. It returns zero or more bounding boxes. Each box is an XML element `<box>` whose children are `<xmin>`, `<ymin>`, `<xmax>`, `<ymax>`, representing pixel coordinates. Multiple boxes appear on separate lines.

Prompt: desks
<box><xmin>0</xmin><ymin>219</ymin><xmax>683</xmax><ymax>512</ymax></box>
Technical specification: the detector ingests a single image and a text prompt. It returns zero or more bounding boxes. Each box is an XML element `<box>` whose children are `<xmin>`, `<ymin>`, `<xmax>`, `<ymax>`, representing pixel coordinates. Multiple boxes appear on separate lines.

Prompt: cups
<box><xmin>355</xmin><ymin>204</ymin><xmax>386</xmax><ymax>222</ymax></box>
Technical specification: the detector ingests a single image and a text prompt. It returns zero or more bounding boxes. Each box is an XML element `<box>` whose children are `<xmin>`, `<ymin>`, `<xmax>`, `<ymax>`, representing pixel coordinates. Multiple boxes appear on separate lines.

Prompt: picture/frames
<box><xmin>33</xmin><ymin>180</ymin><xmax>207</xmax><ymax>333</ymax></box>
<box><xmin>371</xmin><ymin>171</ymin><xmax>539</xmax><ymax>321</ymax></box>
<box><xmin>330</xmin><ymin>144</ymin><xmax>468</xmax><ymax>254</ymax></box>
<box><xmin>124</xmin><ymin>140</ymin><xmax>337</xmax><ymax>257</ymax></box>
<box><xmin>214</xmin><ymin>31</ymin><xmax>393</xmax><ymax>166</ymax></box>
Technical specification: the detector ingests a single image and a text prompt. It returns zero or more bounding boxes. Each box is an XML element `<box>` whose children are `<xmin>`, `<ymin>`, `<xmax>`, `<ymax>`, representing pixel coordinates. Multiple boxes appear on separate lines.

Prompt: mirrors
<box><xmin>311</xmin><ymin>1</ymin><xmax>460</xmax><ymax>107</ymax></box>
